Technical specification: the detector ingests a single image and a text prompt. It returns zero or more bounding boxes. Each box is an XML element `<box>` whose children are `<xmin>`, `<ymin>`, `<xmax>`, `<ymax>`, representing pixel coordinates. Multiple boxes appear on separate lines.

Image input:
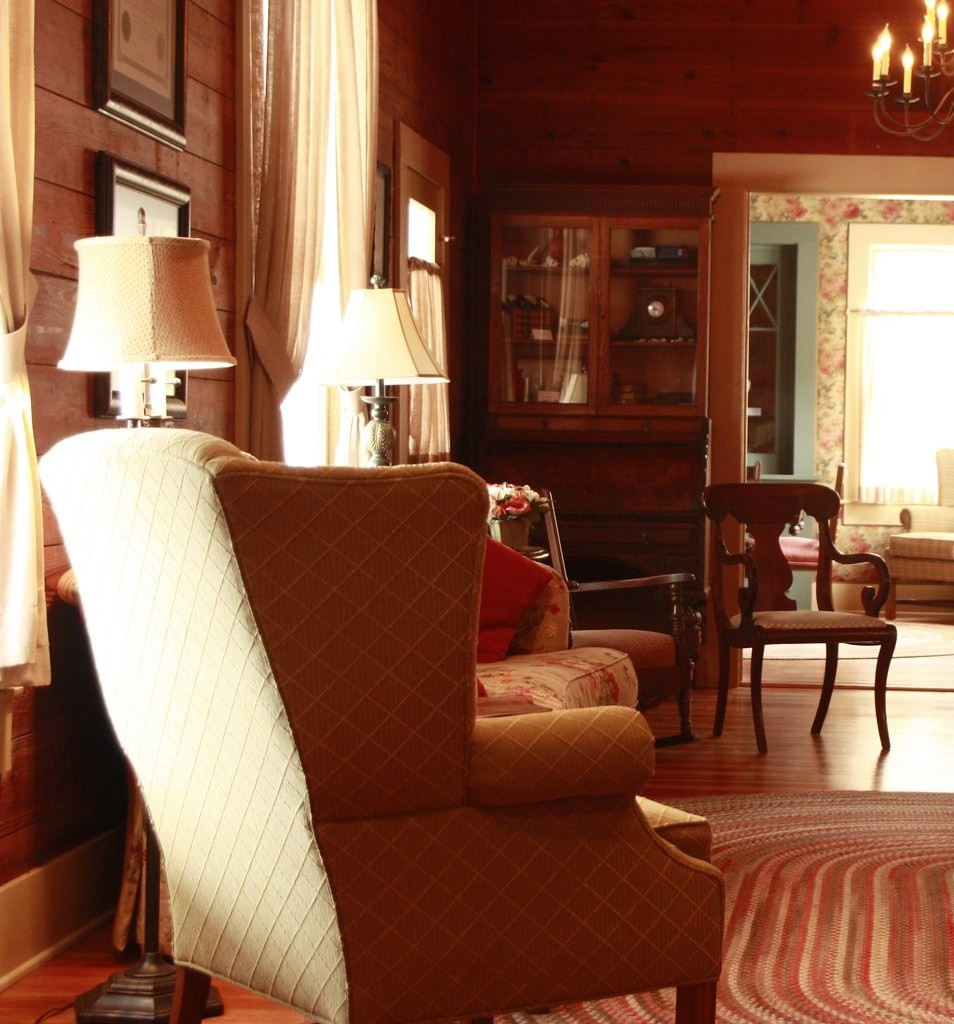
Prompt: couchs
<box><xmin>60</xmin><ymin>485</ymin><xmax>703</xmax><ymax>960</ymax></box>
<box><xmin>39</xmin><ymin>426</ymin><xmax>726</xmax><ymax>1024</ymax></box>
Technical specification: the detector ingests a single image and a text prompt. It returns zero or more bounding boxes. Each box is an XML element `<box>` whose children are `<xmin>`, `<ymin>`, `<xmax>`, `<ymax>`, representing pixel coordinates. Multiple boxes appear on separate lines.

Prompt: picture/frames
<box><xmin>92</xmin><ymin>152</ymin><xmax>192</xmax><ymax>420</ymax></box>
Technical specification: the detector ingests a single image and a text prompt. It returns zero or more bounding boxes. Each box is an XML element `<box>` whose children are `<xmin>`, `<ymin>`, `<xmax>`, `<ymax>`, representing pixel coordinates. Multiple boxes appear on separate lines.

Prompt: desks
<box><xmin>758</xmin><ymin>474</ymin><xmax>819</xmax><ymax>483</ymax></box>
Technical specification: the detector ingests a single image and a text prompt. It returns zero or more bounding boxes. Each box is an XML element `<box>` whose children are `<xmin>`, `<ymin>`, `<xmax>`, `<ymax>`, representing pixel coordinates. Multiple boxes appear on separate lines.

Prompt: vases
<box><xmin>491</xmin><ymin>518</ymin><xmax>532</xmax><ymax>549</ymax></box>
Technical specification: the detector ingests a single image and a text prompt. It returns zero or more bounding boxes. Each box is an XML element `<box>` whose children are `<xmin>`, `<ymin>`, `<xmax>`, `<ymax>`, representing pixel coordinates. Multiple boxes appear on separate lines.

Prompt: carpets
<box><xmin>494</xmin><ymin>787</ymin><xmax>954</xmax><ymax>1024</ymax></box>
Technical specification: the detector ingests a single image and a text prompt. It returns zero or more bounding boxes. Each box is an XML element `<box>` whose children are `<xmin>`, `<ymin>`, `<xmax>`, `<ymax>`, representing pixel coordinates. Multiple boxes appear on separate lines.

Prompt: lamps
<box><xmin>864</xmin><ymin>0</ymin><xmax>954</xmax><ymax>143</ymax></box>
<box><xmin>58</xmin><ymin>208</ymin><xmax>237</xmax><ymax>1024</ymax></box>
<box><xmin>312</xmin><ymin>275</ymin><xmax>451</xmax><ymax>464</ymax></box>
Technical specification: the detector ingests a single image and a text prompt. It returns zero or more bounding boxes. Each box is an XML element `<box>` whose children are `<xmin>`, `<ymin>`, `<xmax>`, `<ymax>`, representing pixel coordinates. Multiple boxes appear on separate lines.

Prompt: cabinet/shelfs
<box><xmin>482</xmin><ymin>180</ymin><xmax>721</xmax><ymax>681</ymax></box>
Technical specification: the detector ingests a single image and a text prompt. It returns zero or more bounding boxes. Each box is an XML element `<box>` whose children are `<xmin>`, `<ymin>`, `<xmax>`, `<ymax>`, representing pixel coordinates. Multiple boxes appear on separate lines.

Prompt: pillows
<box><xmin>476</xmin><ymin>533</ymin><xmax>551</xmax><ymax>664</ymax></box>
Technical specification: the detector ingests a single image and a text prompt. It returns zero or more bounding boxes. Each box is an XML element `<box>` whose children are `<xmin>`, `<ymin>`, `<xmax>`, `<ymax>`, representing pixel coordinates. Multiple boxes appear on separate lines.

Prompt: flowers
<box><xmin>486</xmin><ymin>482</ymin><xmax>557</xmax><ymax>526</ymax></box>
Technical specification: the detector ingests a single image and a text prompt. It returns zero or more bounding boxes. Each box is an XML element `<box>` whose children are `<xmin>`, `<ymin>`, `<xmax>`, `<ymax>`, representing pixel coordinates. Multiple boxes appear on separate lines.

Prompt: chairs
<box><xmin>744</xmin><ymin>463</ymin><xmax>846</xmax><ymax>608</ymax></box>
<box><xmin>883</xmin><ymin>448</ymin><xmax>954</xmax><ymax>620</ymax></box>
<box><xmin>701</xmin><ymin>482</ymin><xmax>897</xmax><ymax>756</ymax></box>
